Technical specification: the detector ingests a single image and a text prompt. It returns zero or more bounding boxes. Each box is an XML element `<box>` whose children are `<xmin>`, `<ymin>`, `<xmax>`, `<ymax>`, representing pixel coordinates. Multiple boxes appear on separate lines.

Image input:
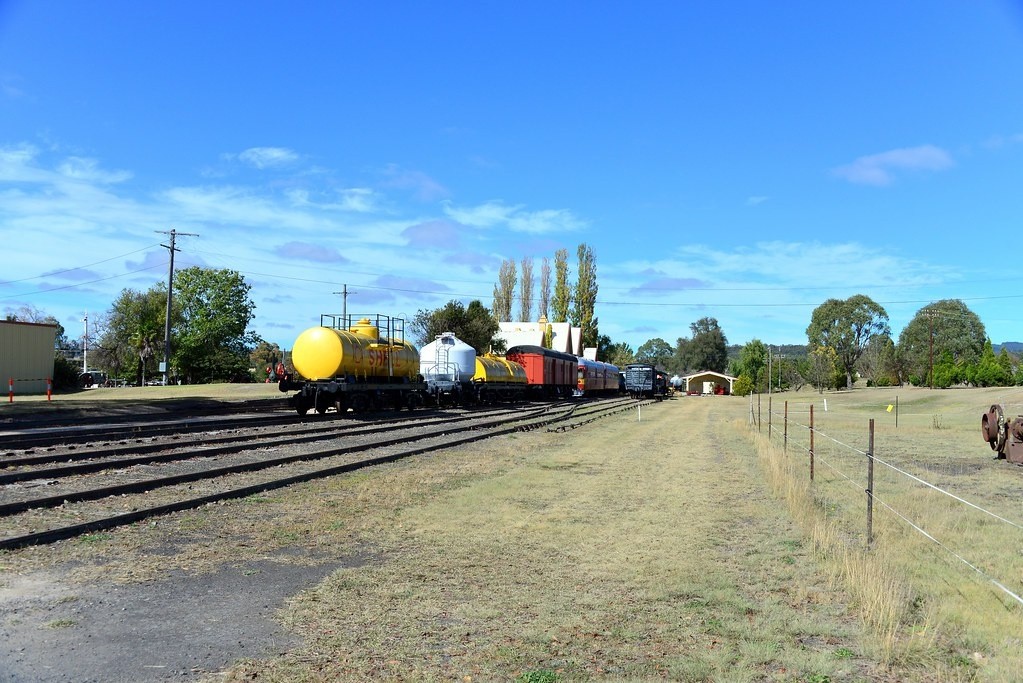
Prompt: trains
<box><xmin>626</xmin><ymin>363</ymin><xmax>676</xmax><ymax>401</ymax></box>
<box><xmin>275</xmin><ymin>283</ymin><xmax>625</xmax><ymax>416</ymax></box>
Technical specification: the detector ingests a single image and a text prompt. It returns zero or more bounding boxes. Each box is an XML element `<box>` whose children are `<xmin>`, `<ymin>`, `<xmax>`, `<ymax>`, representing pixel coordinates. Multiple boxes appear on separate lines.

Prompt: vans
<box><xmin>106</xmin><ymin>379</ymin><xmax>132</xmax><ymax>388</ymax></box>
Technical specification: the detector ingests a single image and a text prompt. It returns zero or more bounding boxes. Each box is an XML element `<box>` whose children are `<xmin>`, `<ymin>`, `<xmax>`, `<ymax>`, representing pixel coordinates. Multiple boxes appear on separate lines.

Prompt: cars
<box><xmin>146</xmin><ymin>378</ymin><xmax>167</xmax><ymax>386</ymax></box>
